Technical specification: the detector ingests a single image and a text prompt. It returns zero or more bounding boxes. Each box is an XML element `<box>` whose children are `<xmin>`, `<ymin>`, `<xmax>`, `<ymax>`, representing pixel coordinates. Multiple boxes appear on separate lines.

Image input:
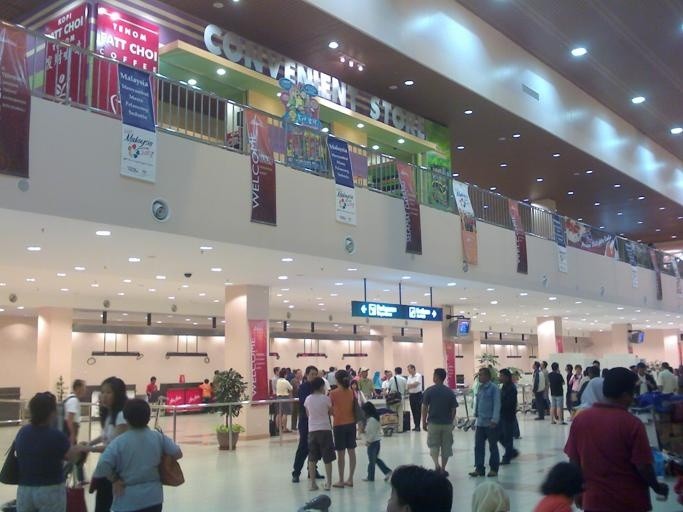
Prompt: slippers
<box><xmin>551</xmin><ymin>420</ymin><xmax>568</xmax><ymax>425</ymax></box>
<box><xmin>308</xmin><ymin>483</ymin><xmax>353</xmax><ymax>490</ymax></box>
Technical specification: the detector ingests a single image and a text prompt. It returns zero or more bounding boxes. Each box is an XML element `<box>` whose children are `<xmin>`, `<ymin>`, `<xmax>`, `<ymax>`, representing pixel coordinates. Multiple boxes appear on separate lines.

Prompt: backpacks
<box><xmin>56</xmin><ymin>396</ymin><xmax>79</xmax><ymax>433</ymax></box>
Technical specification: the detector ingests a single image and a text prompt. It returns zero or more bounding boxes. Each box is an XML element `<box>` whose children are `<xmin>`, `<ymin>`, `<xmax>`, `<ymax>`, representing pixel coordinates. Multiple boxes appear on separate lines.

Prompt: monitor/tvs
<box><xmin>632</xmin><ymin>332</ymin><xmax>644</xmax><ymax>343</ymax></box>
<box><xmin>449</xmin><ymin>320</ymin><xmax>471</xmax><ymax>337</ymax></box>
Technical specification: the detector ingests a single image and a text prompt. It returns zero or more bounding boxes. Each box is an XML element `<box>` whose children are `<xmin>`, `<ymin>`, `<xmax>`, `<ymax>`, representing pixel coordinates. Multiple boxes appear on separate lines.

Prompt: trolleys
<box><xmin>518</xmin><ymin>382</ymin><xmax>532</xmax><ymax>413</ymax></box>
<box><xmin>0</xmin><ymin>441</ymin><xmax>87</xmax><ymax>512</ymax></box>
<box><xmin>457</xmin><ymin>388</ymin><xmax>474</xmax><ymax>431</ymax></box>
<box><xmin>377</xmin><ymin>408</ymin><xmax>404</xmax><ymax>438</ymax></box>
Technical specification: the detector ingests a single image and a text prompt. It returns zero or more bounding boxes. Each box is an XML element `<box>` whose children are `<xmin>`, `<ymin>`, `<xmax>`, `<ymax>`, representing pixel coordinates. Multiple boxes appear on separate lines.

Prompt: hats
<box><xmin>360</xmin><ymin>366</ymin><xmax>370</xmax><ymax>373</ymax></box>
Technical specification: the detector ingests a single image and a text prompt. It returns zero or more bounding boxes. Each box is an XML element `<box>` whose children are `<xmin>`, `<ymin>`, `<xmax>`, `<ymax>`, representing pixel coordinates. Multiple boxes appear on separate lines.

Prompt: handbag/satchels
<box><xmin>0</xmin><ymin>442</ymin><xmax>21</xmax><ymax>486</ymax></box>
<box><xmin>154</xmin><ymin>452</ymin><xmax>186</xmax><ymax>487</ymax></box>
<box><xmin>384</xmin><ymin>389</ymin><xmax>402</xmax><ymax>405</ymax></box>
<box><xmin>67</xmin><ymin>485</ymin><xmax>87</xmax><ymax>511</ymax></box>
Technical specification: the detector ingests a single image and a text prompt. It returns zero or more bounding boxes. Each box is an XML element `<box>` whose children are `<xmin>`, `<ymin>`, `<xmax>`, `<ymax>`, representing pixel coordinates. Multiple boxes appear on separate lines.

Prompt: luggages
<box><xmin>403</xmin><ymin>393</ymin><xmax>411</xmax><ymax>433</ymax></box>
<box><xmin>379</xmin><ymin>412</ymin><xmax>401</xmax><ymax>425</ymax></box>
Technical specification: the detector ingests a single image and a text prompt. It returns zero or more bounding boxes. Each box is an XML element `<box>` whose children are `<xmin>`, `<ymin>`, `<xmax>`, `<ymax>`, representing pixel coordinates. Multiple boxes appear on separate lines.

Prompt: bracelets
<box><xmin>91</xmin><ymin>443</ymin><xmax>97</xmax><ymax>452</ymax></box>
<box><xmin>110</xmin><ymin>474</ymin><xmax>118</xmax><ymax>485</ymax></box>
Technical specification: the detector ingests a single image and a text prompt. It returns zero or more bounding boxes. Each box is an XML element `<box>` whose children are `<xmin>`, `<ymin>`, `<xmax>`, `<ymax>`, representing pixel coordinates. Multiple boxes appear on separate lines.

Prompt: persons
<box><xmin>469</xmin><ymin>367</ymin><xmax>520</xmax><ymax>476</ymax></box>
<box><xmin>471</xmin><ymin>482</ymin><xmax>511</xmax><ymax>511</ymax></box>
<box><xmin>385</xmin><ymin>464</ymin><xmax>452</xmax><ymax>512</ymax></box>
<box><xmin>629</xmin><ymin>362</ymin><xmax>679</xmax><ymax>397</ymax></box>
<box><xmin>197</xmin><ymin>378</ymin><xmax>211</xmax><ymax>413</ymax></box>
<box><xmin>533</xmin><ymin>461</ymin><xmax>591</xmax><ymax>512</ymax></box>
<box><xmin>91</xmin><ymin>399</ymin><xmax>183</xmax><ymax>511</ymax></box>
<box><xmin>532</xmin><ymin>360</ymin><xmax>600</xmax><ymax>425</ymax></box>
<box><xmin>563</xmin><ymin>366</ymin><xmax>669</xmax><ymax>511</ymax></box>
<box><xmin>145</xmin><ymin>376</ymin><xmax>157</xmax><ymax>398</ymax></box>
<box><xmin>423</xmin><ymin>368</ymin><xmax>458</xmax><ymax>477</ymax></box>
<box><xmin>273</xmin><ymin>365</ymin><xmax>423</xmax><ymax>490</ymax></box>
<box><xmin>12</xmin><ymin>390</ymin><xmax>87</xmax><ymax>511</ymax></box>
<box><xmin>74</xmin><ymin>376</ymin><xmax>130</xmax><ymax>511</ymax></box>
<box><xmin>61</xmin><ymin>379</ymin><xmax>89</xmax><ymax>486</ymax></box>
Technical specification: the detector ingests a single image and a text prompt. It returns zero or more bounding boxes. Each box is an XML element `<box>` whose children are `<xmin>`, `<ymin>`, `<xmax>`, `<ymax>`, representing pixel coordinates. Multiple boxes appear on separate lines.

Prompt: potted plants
<box><xmin>211</xmin><ymin>368</ymin><xmax>249</xmax><ymax>450</ymax></box>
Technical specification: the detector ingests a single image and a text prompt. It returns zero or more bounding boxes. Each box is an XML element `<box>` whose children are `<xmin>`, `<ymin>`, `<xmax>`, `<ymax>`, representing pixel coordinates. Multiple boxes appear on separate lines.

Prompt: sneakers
<box><xmin>435</xmin><ymin>469</ymin><xmax>449</xmax><ymax>477</ymax></box>
<box><xmin>362</xmin><ymin>470</ymin><xmax>392</xmax><ymax>482</ymax></box>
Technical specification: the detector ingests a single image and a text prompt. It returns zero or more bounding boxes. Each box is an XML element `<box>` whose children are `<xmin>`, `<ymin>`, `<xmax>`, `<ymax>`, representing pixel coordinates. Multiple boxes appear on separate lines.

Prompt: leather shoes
<box><xmin>468</xmin><ymin>469</ymin><xmax>485</xmax><ymax>476</ymax></box>
<box><xmin>308</xmin><ymin>469</ymin><xmax>325</xmax><ymax>479</ymax></box>
<box><xmin>282</xmin><ymin>430</ymin><xmax>293</xmax><ymax>432</ymax></box>
<box><xmin>535</xmin><ymin>416</ymin><xmax>544</xmax><ymax>420</ymax></box>
<box><xmin>292</xmin><ymin>470</ymin><xmax>301</xmax><ymax>482</ymax></box>
<box><xmin>487</xmin><ymin>469</ymin><xmax>498</xmax><ymax>477</ymax></box>
